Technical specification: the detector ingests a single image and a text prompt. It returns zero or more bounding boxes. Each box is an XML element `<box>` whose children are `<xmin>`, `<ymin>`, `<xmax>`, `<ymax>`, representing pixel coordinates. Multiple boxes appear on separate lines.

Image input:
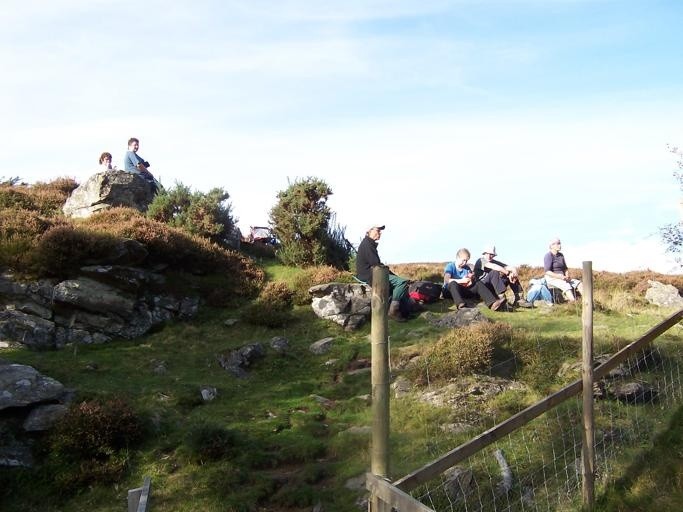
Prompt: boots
<box><xmin>387</xmin><ymin>300</ymin><xmax>416</xmax><ymax>323</ymax></box>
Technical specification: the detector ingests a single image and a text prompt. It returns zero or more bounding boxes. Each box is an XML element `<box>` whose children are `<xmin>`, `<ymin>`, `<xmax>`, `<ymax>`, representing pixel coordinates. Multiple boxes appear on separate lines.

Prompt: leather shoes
<box><xmin>490</xmin><ymin>298</ymin><xmax>507</xmax><ymax>311</ymax></box>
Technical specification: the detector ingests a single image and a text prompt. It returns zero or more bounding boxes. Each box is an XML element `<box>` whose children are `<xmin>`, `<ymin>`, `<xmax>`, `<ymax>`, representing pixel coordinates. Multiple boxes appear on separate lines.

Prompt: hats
<box><xmin>481</xmin><ymin>244</ymin><xmax>498</xmax><ymax>256</ymax></box>
<box><xmin>366</xmin><ymin>223</ymin><xmax>386</xmax><ymax>234</ymax></box>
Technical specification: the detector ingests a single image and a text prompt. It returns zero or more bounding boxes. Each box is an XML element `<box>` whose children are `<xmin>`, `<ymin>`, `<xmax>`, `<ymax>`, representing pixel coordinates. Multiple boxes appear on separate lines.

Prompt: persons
<box><xmin>98</xmin><ymin>137</ymin><xmax>167</xmax><ymax>198</ymax></box>
<box><xmin>544</xmin><ymin>237</ymin><xmax>583</xmax><ymax>304</ymax></box>
<box><xmin>441</xmin><ymin>238</ymin><xmax>533</xmax><ymax>311</ymax></box>
<box><xmin>355</xmin><ymin>223</ymin><xmax>417</xmax><ymax>323</ymax></box>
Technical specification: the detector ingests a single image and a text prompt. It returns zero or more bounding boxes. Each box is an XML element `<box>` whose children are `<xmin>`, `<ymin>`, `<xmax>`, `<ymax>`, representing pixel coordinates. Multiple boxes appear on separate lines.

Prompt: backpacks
<box><xmin>407</xmin><ymin>281</ymin><xmax>442</xmax><ymax>304</ymax></box>
